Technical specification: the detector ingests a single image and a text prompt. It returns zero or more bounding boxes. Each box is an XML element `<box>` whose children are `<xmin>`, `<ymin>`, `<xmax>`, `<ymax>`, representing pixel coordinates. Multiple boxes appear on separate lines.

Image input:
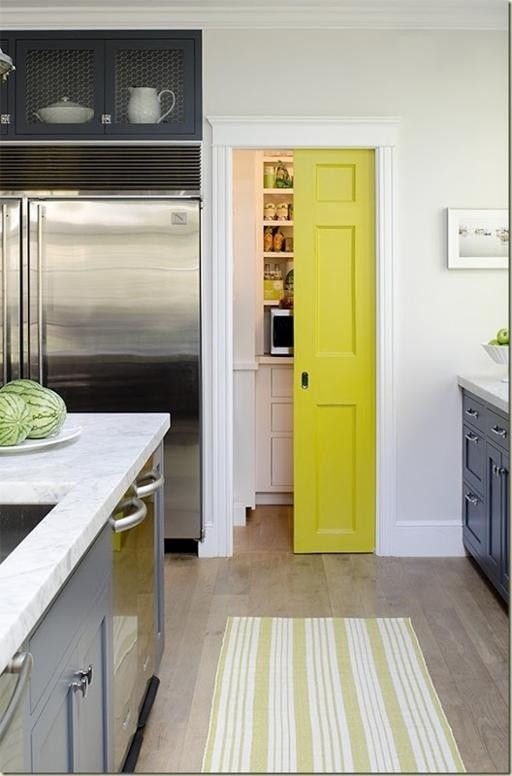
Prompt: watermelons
<box><xmin>0</xmin><ymin>379</ymin><xmax>67</xmax><ymax>448</ymax></box>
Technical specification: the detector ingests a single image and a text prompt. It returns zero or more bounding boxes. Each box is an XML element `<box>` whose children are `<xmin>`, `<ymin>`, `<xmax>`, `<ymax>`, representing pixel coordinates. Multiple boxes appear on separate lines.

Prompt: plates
<box><xmin>0</xmin><ymin>419</ymin><xmax>86</xmax><ymax>456</ymax></box>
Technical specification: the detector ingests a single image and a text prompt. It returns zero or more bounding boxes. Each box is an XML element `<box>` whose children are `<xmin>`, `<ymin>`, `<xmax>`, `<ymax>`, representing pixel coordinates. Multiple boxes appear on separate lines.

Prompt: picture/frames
<box><xmin>446</xmin><ymin>204</ymin><xmax>509</xmax><ymax>270</ymax></box>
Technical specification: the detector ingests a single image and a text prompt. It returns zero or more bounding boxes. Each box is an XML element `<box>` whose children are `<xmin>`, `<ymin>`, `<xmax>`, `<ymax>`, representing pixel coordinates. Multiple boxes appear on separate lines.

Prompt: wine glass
<box><xmin>480</xmin><ymin>343</ymin><xmax>508</xmax><ymax>383</ymax></box>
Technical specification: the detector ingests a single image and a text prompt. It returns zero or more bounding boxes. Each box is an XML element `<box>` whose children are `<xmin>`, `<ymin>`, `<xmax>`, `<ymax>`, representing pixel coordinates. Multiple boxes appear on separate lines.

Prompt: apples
<box><xmin>488</xmin><ymin>329</ymin><xmax>509</xmax><ymax>346</ymax></box>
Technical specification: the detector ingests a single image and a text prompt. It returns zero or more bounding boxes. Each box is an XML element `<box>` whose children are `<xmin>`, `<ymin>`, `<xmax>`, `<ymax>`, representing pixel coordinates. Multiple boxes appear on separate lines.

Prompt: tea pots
<box><xmin>124</xmin><ymin>85</ymin><xmax>177</xmax><ymax>125</ymax></box>
<box><xmin>30</xmin><ymin>96</ymin><xmax>96</xmax><ymax>125</ymax></box>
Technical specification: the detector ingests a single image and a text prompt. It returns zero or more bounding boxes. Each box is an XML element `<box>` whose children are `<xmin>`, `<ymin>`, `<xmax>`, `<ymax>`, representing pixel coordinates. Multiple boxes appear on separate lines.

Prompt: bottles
<box><xmin>263</xmin><ymin>263</ymin><xmax>283</xmax><ymax>301</ymax></box>
<box><xmin>264</xmin><ymin>165</ymin><xmax>276</xmax><ymax>187</ymax></box>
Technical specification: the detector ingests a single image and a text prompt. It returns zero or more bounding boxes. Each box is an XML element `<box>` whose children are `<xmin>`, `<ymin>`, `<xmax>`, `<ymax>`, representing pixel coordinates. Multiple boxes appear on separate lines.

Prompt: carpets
<box><xmin>200</xmin><ymin>616</ymin><xmax>469</xmax><ymax>773</ymax></box>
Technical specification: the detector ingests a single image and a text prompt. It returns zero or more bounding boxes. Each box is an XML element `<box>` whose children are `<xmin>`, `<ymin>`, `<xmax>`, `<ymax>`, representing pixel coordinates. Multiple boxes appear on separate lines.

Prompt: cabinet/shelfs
<box><xmin>0</xmin><ymin>28</ymin><xmax>202</xmax><ymax>138</ymax></box>
<box><xmin>254</xmin><ymin>149</ymin><xmax>293</xmax><ymax>504</ymax></box>
<box><xmin>462</xmin><ymin>388</ymin><xmax>510</xmax><ymax>611</ymax></box>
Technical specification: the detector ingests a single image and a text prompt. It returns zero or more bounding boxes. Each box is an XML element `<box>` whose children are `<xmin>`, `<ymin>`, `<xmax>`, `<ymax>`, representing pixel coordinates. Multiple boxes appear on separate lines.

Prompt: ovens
<box><xmin>0</xmin><ymin>187</ymin><xmax>209</xmax><ymax>545</ymax></box>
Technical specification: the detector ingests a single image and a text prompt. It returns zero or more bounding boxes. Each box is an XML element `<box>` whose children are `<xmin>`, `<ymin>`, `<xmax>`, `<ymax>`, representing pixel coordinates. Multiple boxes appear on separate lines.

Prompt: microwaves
<box><xmin>267</xmin><ymin>306</ymin><xmax>297</xmax><ymax>358</ymax></box>
